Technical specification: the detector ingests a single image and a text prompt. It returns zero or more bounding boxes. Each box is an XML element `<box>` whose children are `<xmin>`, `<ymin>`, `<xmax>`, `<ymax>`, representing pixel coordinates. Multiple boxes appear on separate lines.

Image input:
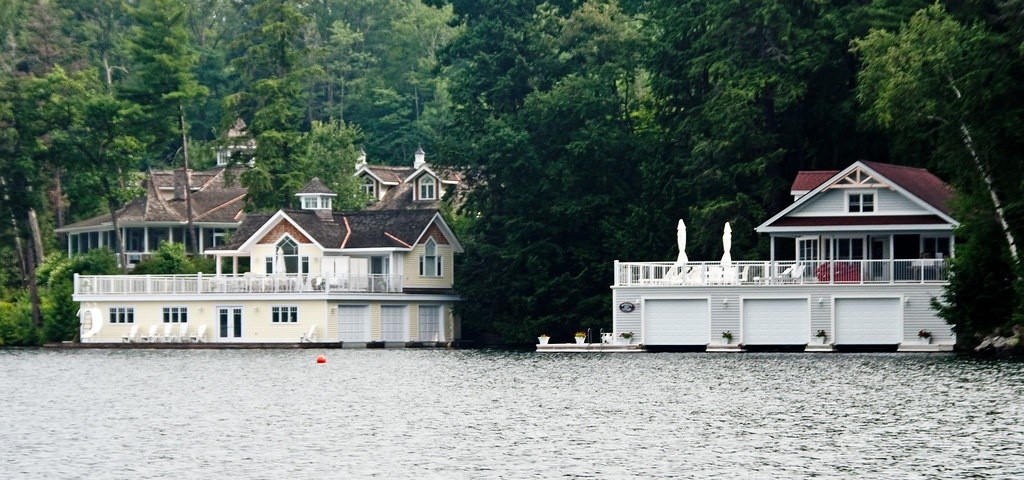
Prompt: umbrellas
<box><xmin>276</xmin><ymin>247</ymin><xmax>286</xmax><ymax>274</ymax></box>
<box><xmin>676</xmin><ymin>219</ymin><xmax>688</xmax><ymax>267</ymax></box>
<box><xmin>720</xmin><ymin>222</ymin><xmax>732</xmax><ymax>268</ymax></box>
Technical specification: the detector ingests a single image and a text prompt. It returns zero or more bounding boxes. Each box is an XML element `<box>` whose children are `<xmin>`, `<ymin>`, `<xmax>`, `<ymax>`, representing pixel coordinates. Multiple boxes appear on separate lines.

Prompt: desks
<box><xmin>911</xmin><ymin>259</ymin><xmax>947</xmax><ymax>280</ymax></box>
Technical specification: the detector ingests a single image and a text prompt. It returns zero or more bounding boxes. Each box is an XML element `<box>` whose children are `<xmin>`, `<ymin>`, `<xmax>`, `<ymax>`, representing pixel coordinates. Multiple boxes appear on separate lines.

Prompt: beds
<box><xmin>82</xmin><ymin>327</ymin><xmax>101</xmax><ymax>342</ymax></box>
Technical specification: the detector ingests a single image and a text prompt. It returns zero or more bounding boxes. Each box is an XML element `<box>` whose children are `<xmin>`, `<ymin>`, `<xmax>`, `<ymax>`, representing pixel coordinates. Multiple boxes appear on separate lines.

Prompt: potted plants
<box><xmin>917</xmin><ymin>329</ymin><xmax>931</xmax><ymax>345</ymax></box>
<box><xmin>538</xmin><ymin>335</ymin><xmax>550</xmax><ymax>344</ymax></box>
<box><xmin>619</xmin><ymin>332</ymin><xmax>634</xmax><ymax>344</ymax></box>
<box><xmin>575</xmin><ymin>332</ymin><xmax>586</xmax><ymax>344</ymax></box>
<box><xmin>815</xmin><ymin>330</ymin><xmax>826</xmax><ymax>344</ymax></box>
<box><xmin>722</xmin><ymin>331</ymin><xmax>732</xmax><ymax>345</ymax></box>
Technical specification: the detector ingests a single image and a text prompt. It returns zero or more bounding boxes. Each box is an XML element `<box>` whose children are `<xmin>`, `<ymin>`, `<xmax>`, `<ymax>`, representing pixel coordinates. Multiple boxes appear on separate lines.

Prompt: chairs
<box><xmin>638</xmin><ymin>265</ymin><xmax>749</xmax><ymax>286</ymax></box>
<box><xmin>122</xmin><ymin>324</ymin><xmax>207</xmax><ymax>343</ymax></box>
<box><xmin>753</xmin><ymin>265</ymin><xmax>806</xmax><ymax>285</ymax></box>
<box><xmin>300</xmin><ymin>326</ymin><xmax>316</xmax><ymax>342</ymax></box>
